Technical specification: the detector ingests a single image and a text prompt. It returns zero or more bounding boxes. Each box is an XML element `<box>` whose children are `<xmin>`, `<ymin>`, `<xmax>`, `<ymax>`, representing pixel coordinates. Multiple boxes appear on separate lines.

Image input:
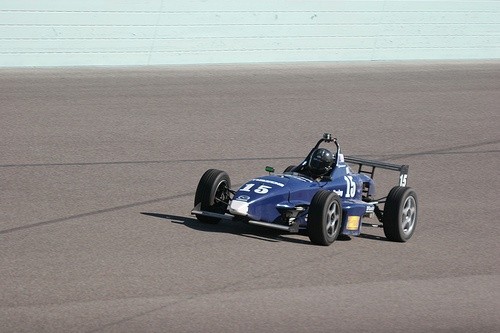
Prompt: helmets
<box><xmin>309</xmin><ymin>148</ymin><xmax>333</xmax><ymax>176</ymax></box>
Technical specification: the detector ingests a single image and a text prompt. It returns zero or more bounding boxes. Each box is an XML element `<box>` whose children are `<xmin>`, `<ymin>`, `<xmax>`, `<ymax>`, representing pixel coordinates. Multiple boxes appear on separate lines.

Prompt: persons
<box><xmin>309</xmin><ymin>148</ymin><xmax>333</xmax><ymax>179</ymax></box>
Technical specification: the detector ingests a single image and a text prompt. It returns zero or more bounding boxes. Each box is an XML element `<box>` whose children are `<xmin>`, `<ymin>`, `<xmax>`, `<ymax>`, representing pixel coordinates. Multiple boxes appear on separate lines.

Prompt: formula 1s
<box><xmin>190</xmin><ymin>133</ymin><xmax>418</xmax><ymax>246</ymax></box>
